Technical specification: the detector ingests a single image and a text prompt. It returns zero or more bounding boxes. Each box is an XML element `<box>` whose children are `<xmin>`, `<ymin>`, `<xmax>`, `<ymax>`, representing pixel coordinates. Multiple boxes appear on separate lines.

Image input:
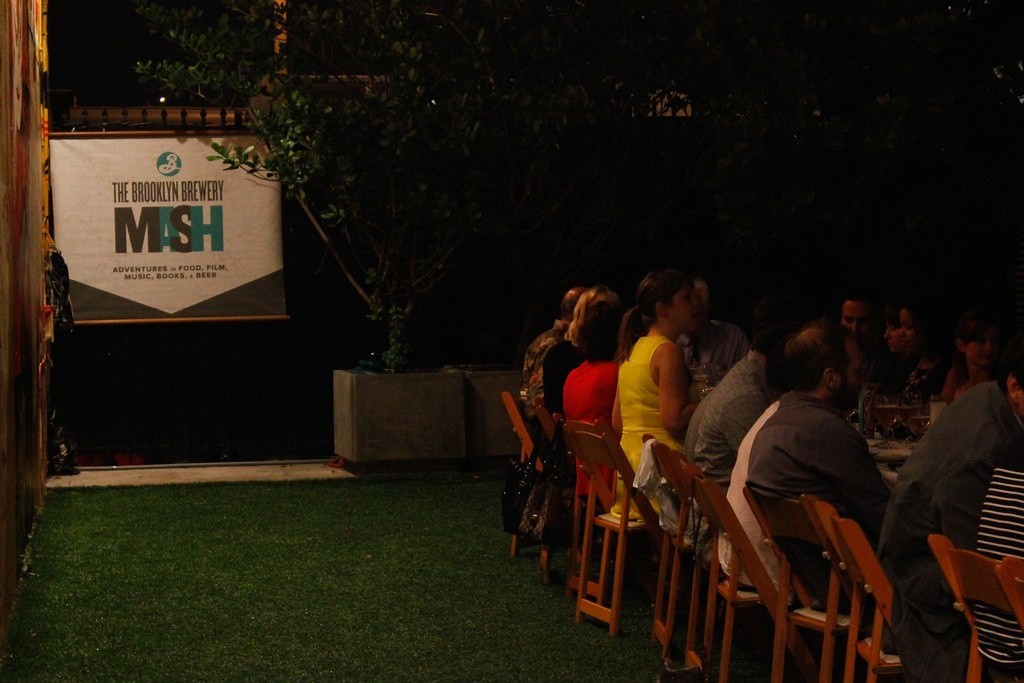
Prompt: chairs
<box><xmin>643</xmin><ymin>434</ymin><xmax>696</xmax><ymax>659</ymax></box>
<box><xmin>500</xmin><ymin>391</ymin><xmax>573</xmax><ymax>569</ymax></box>
<box><xmin>927</xmin><ymin>534</ymin><xmax>1024</xmax><ymax>683</ymax></box>
<box><xmin>802</xmin><ymin>494</ymin><xmax>852</xmax><ymax>683</ymax></box>
<box><xmin>563</xmin><ymin>421</ymin><xmax>650</xmax><ymax>606</ymax></box>
<box><xmin>694</xmin><ymin>474</ymin><xmax>819</xmax><ymax>683</ymax></box>
<box><xmin>815</xmin><ymin>501</ymin><xmax>903</xmax><ymax>683</ymax></box>
<box><xmin>575</xmin><ymin>419</ymin><xmax>699</xmax><ymax>637</ymax></box>
<box><xmin>532</xmin><ymin>396</ymin><xmax>606</xmax><ymax>584</ymax></box>
<box><xmin>669</xmin><ymin>450</ymin><xmax>740</xmax><ymax>683</ymax></box>
<box><xmin>743</xmin><ymin>486</ymin><xmax>854</xmax><ymax>683</ymax></box>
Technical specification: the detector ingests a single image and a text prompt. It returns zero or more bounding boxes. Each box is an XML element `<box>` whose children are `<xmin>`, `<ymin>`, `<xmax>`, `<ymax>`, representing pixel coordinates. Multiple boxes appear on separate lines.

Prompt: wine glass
<box><xmin>690</xmin><ymin>366</ymin><xmax>709</xmax><ymax>404</ymax></box>
<box><xmin>898</xmin><ymin>392</ymin><xmax>921</xmax><ymax>447</ymax></box>
<box><xmin>872</xmin><ymin>394</ymin><xmax>900</xmax><ymax>449</ymax></box>
<box><xmin>908</xmin><ymin>398</ymin><xmax>932</xmax><ymax>451</ymax></box>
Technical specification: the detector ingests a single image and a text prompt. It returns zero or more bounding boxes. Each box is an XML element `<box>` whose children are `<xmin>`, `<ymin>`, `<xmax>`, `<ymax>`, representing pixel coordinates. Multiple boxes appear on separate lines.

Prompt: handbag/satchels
<box><xmin>520</xmin><ymin>456</ymin><xmax>574</xmax><ymax>547</ymax></box>
<box><xmin>499</xmin><ymin>458</ymin><xmax>538</xmax><ymax>535</ymax></box>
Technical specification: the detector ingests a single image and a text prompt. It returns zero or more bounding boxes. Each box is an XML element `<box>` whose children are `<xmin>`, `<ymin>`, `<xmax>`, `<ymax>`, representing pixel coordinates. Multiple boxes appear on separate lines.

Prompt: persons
<box><xmin>825</xmin><ymin>295</ymin><xmax>1023</xmax><ymax>435</ymax></box>
<box><xmin>870</xmin><ymin>337</ymin><xmax>1023</xmax><ymax>683</ymax></box>
<box><xmin>692</xmin><ymin>294</ymin><xmax>828</xmax><ymax>568</ymax></box>
<box><xmin>745</xmin><ymin>321</ymin><xmax>895</xmax><ymax>619</ymax></box>
<box><xmin>527</xmin><ymin>266</ymin><xmax>779</xmax><ymax>524</ymax></box>
<box><xmin>972</xmin><ymin>435</ymin><xmax>1024</xmax><ymax>683</ymax></box>
